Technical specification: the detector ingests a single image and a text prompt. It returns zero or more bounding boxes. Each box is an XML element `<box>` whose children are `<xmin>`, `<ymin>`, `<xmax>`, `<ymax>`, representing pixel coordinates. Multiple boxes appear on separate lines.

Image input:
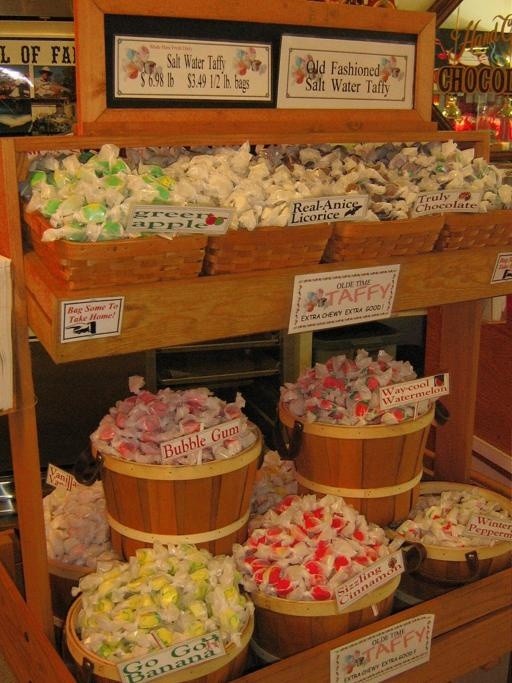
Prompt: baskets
<box><xmin>322</xmin><ymin>210</ymin><xmax>445</xmax><ymax>262</ymax></box>
<box><xmin>207</xmin><ymin>225</ymin><xmax>336</xmax><ymax>278</ymax></box>
<box><xmin>19</xmin><ymin>198</ymin><xmax>211</xmax><ymax>290</ymax></box>
<box><xmin>432</xmin><ymin>208</ymin><xmax>511</xmax><ymax>250</ymax></box>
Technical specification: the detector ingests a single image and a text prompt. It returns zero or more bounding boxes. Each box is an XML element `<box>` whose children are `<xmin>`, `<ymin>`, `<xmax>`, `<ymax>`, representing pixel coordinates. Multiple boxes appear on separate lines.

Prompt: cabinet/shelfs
<box><xmin>1</xmin><ymin>132</ymin><xmax>511</xmax><ymax>683</ymax></box>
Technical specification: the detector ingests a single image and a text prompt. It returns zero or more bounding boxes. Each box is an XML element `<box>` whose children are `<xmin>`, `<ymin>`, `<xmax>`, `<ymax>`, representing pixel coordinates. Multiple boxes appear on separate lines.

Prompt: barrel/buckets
<box><xmin>386</xmin><ymin>480</ymin><xmax>512</xmax><ymax>612</ymax></box>
<box><xmin>251</xmin><ymin>573</ymin><xmax>402</xmax><ymax>664</ymax></box>
<box><xmin>101</xmin><ymin>419</ymin><xmax>264</xmax><ymax>563</ymax></box>
<box><xmin>271</xmin><ymin>400</ymin><xmax>452</xmax><ymax>528</ymax></box>
<box><xmin>64</xmin><ymin>595</ymin><xmax>254</xmax><ymax>682</ymax></box>
<box><xmin>47</xmin><ymin>561</ymin><xmax>93</xmax><ymax>631</ymax></box>
